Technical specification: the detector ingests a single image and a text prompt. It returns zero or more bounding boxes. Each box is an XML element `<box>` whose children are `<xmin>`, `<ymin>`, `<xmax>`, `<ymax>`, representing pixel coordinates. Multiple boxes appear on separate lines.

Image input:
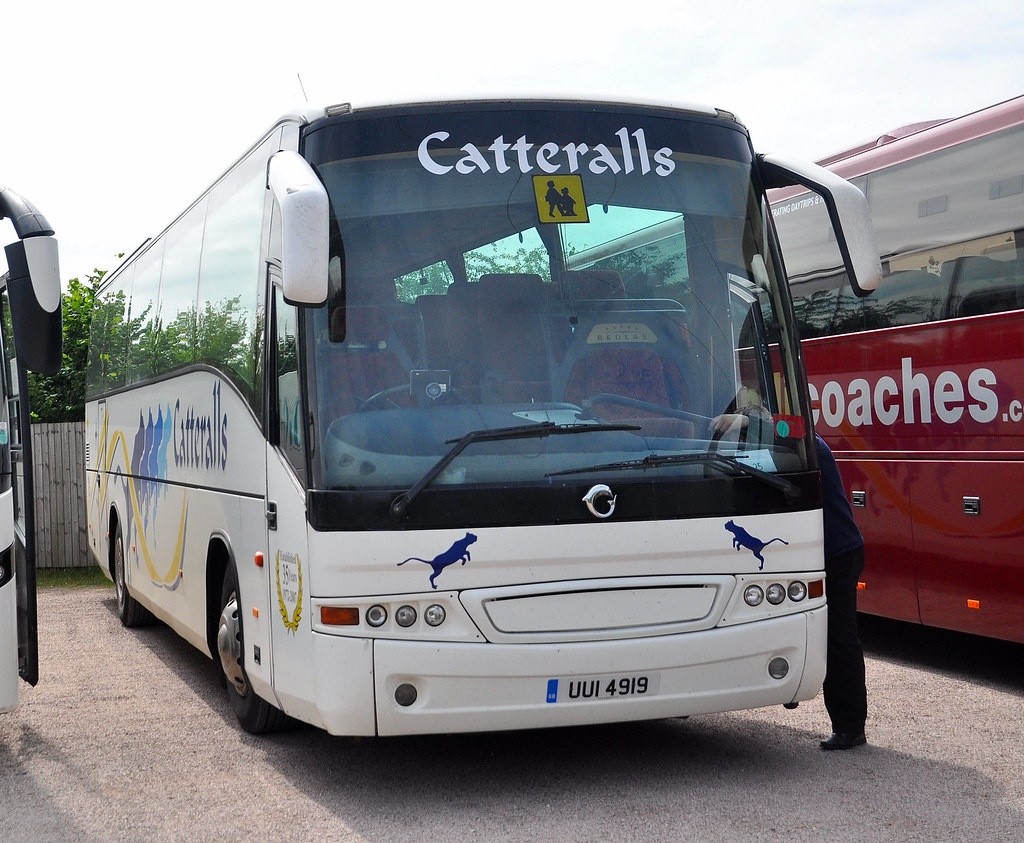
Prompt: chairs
<box><xmin>882</xmin><ymin>256</ymin><xmax>1018</xmax><ymax>325</ymax></box>
<box><xmin>328</xmin><ymin>266</ymin><xmax>695</xmax><ymax>439</ymax></box>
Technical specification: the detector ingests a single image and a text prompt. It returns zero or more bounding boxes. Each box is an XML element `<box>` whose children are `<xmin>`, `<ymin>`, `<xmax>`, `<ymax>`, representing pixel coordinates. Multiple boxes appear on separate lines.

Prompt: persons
<box><xmin>708</xmin><ymin>404</ymin><xmax>867</xmax><ymax>750</ymax></box>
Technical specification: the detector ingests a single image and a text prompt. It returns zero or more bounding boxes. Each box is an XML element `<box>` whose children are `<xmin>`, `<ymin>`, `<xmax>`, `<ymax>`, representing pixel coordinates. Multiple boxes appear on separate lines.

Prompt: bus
<box><xmin>80</xmin><ymin>97</ymin><xmax>885</xmax><ymax>746</ymax></box>
<box><xmin>1</xmin><ymin>190</ymin><xmax>65</xmax><ymax>717</ymax></box>
<box><xmin>571</xmin><ymin>94</ymin><xmax>1024</xmax><ymax>638</ymax></box>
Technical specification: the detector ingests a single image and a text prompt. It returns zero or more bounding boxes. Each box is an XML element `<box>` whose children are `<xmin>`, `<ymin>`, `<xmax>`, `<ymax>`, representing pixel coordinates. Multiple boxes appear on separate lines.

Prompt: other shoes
<box><xmin>820</xmin><ymin>730</ymin><xmax>867</xmax><ymax>751</ymax></box>
